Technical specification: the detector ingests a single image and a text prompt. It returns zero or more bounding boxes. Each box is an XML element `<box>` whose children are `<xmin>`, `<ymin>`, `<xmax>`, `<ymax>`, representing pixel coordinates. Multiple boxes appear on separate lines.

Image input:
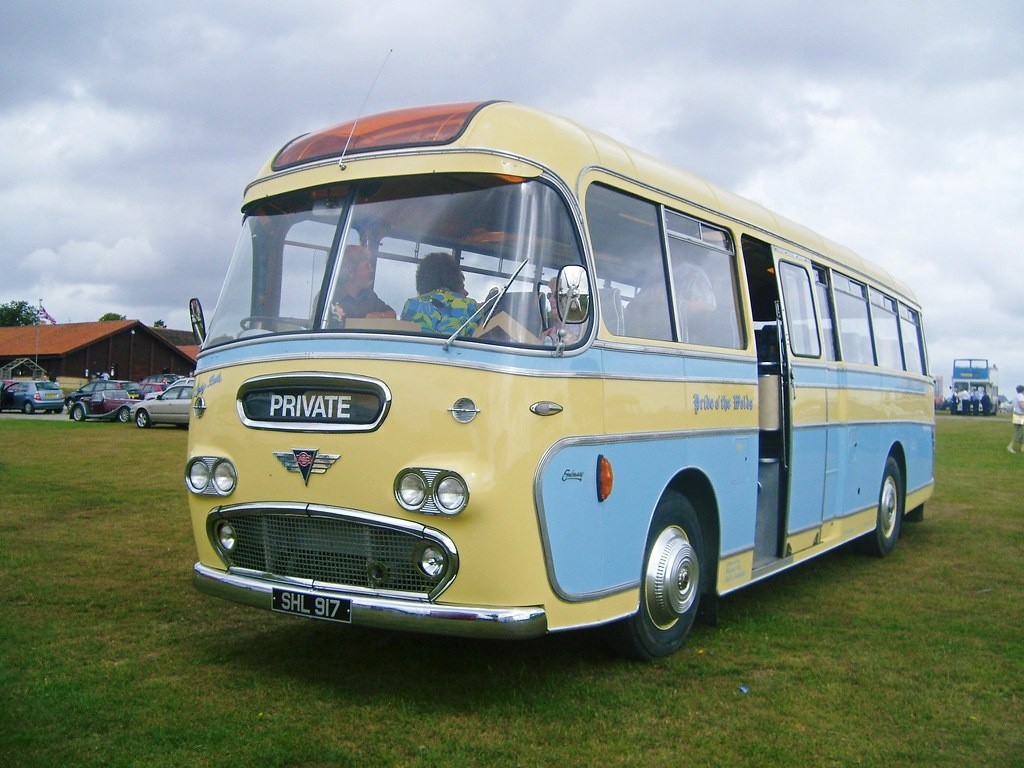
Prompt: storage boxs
<box><xmin>472</xmin><ymin>311</ymin><xmax>542</xmax><ymax>345</ymax></box>
<box><xmin>344</xmin><ymin>317</ymin><xmax>421</xmax><ymax>330</ymax></box>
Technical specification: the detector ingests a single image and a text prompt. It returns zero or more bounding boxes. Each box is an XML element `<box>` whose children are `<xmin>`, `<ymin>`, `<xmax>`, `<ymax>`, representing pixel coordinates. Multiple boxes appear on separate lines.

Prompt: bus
<box><xmin>184</xmin><ymin>98</ymin><xmax>935</xmax><ymax>665</ymax></box>
<box><xmin>950</xmin><ymin>358</ymin><xmax>1001</xmax><ymax>417</ymax></box>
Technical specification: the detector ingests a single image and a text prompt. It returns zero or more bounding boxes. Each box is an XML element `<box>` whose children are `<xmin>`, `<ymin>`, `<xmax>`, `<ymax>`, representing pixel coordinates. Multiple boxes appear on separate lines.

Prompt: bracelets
<box><xmin>381</xmin><ymin>311</ymin><xmax>385</xmax><ymax>318</ymax></box>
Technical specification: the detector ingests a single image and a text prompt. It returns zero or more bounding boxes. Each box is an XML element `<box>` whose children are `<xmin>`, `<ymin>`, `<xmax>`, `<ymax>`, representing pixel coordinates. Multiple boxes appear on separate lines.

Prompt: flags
<box><xmin>37</xmin><ymin>305</ymin><xmax>57</xmax><ymax>326</ymax></box>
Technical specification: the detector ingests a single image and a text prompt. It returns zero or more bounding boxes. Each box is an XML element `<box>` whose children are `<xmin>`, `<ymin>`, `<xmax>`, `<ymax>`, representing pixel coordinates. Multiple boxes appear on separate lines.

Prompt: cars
<box><xmin>0</xmin><ymin>379</ymin><xmax>66</xmax><ymax>415</ymax></box>
<box><xmin>68</xmin><ymin>388</ymin><xmax>144</xmax><ymax>423</ymax></box>
<box><xmin>133</xmin><ymin>384</ymin><xmax>195</xmax><ymax>429</ymax></box>
<box><xmin>140</xmin><ymin>372</ymin><xmax>196</xmax><ymax>402</ymax></box>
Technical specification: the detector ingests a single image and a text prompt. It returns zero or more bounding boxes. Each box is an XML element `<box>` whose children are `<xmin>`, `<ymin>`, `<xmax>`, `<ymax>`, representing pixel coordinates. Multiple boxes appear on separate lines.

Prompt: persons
<box><xmin>950</xmin><ymin>387</ymin><xmax>993</xmax><ymax>416</ymax></box>
<box><xmin>624</xmin><ymin>266</ymin><xmax>721</xmax><ymax>344</ymax></box>
<box><xmin>95</xmin><ymin>362</ymin><xmax>117</xmax><ymax>380</ymax></box>
<box><xmin>1006</xmin><ymin>384</ymin><xmax>1024</xmax><ymax>454</ymax></box>
<box><xmin>309</xmin><ymin>245</ymin><xmax>397</xmax><ymax>333</ymax></box>
<box><xmin>43</xmin><ymin>372</ymin><xmax>56</xmax><ymax>414</ymax></box>
<box><xmin>400</xmin><ymin>253</ymin><xmax>483</xmax><ymax>342</ymax></box>
<box><xmin>538</xmin><ymin>274</ymin><xmax>584</xmax><ymax>349</ymax></box>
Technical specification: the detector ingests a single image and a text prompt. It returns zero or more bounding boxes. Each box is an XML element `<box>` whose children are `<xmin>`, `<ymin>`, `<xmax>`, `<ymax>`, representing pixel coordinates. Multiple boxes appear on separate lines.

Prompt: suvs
<box><xmin>64</xmin><ymin>379</ymin><xmax>147</xmax><ymax>414</ymax></box>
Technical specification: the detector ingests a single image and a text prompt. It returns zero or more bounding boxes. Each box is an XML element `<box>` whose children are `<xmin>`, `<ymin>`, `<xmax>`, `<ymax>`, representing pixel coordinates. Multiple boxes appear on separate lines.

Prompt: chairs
<box><xmin>482</xmin><ymin>293</ymin><xmax>546</xmax><ymax>334</ymax></box>
